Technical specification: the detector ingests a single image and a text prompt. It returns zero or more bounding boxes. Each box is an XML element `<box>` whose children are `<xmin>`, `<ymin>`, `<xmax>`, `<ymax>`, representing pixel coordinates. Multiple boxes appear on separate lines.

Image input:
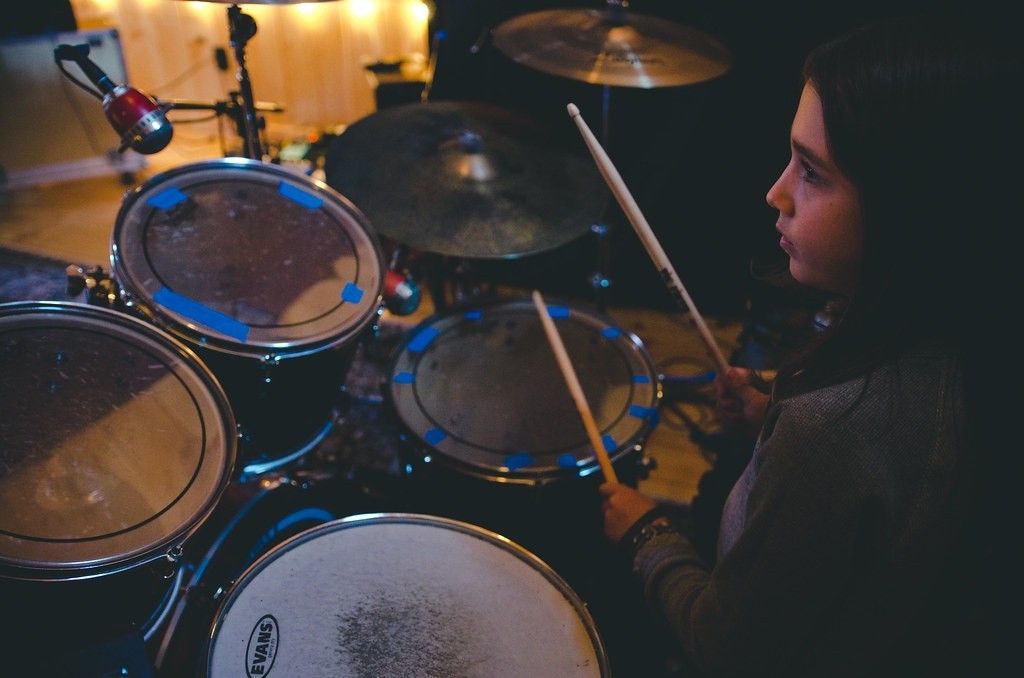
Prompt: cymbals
<box><xmin>321</xmin><ymin>102</ymin><xmax>614</xmax><ymax>261</ymax></box>
<box><xmin>490</xmin><ymin>6</ymin><xmax>736</xmax><ymax>88</ymax></box>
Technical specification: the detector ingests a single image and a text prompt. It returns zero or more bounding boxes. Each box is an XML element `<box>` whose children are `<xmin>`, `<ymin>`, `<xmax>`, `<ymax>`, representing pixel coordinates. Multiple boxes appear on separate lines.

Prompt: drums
<box><xmin>112</xmin><ymin>155</ymin><xmax>385</xmax><ymax>483</ymax></box>
<box><xmin>0</xmin><ymin>300</ymin><xmax>239</xmax><ymax>645</ymax></box>
<box><xmin>388</xmin><ymin>295</ymin><xmax>664</xmax><ymax>554</ymax></box>
<box><xmin>198</xmin><ymin>510</ymin><xmax>612</xmax><ymax>678</ymax></box>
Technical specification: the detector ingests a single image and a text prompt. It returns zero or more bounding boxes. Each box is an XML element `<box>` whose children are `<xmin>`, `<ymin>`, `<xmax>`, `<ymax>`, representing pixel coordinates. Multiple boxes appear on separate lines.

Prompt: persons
<box><xmin>599</xmin><ymin>19</ymin><xmax>1024</xmax><ymax>678</ymax></box>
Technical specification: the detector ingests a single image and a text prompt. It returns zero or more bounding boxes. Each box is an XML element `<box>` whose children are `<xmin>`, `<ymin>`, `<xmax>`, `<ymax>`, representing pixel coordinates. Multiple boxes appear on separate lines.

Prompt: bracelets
<box><xmin>618</xmin><ymin>508</ymin><xmax>678</xmax><ymax>558</ymax></box>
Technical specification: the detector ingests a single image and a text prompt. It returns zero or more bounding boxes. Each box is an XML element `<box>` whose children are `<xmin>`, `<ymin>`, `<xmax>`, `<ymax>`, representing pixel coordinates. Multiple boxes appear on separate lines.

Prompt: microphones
<box><xmin>55</xmin><ymin>43</ymin><xmax>173</xmax><ymax>155</ymax></box>
<box><xmin>227</xmin><ymin>5</ymin><xmax>257</xmax><ymax>41</ymax></box>
<box><xmin>381</xmin><ymin>271</ymin><xmax>420</xmax><ymax>316</ymax></box>
